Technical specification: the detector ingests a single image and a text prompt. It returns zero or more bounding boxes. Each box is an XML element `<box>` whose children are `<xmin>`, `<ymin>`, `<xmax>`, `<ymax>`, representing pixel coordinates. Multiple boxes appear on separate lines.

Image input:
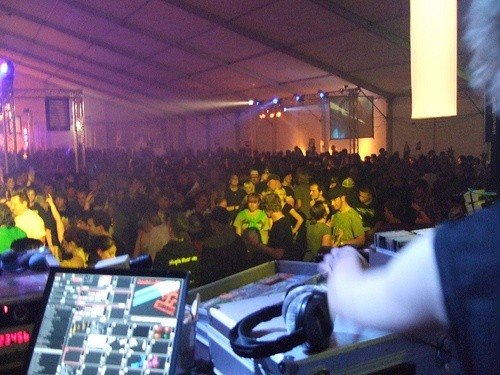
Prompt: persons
<box><xmin>1</xmin><ymin>145</ymin><xmax>499</xmax><ymax>288</ymax></box>
<box><xmin>318</xmin><ymin>0</ymin><xmax>499</xmax><ymax>374</ymax></box>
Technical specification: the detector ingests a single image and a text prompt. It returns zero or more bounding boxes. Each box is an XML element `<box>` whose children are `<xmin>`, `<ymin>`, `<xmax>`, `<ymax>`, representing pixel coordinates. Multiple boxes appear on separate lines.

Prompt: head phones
<box><xmin>229</xmin><ymin>282</ymin><xmax>332</xmax><ymax>359</ymax></box>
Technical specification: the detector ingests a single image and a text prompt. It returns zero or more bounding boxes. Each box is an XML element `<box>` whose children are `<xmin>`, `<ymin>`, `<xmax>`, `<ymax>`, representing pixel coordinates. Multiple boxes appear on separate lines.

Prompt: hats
<box><xmin>329</xmin><ymin>188</ymin><xmax>347</xmax><ymax>200</ymax></box>
<box><xmin>265</xmin><ymin>174</ymin><xmax>281</xmax><ymax>183</ymax></box>
<box><xmin>207</xmin><ymin>206</ymin><xmax>230</xmax><ymax>224</ymax></box>
<box><xmin>249</xmin><ymin>169</ymin><xmax>259</xmax><ymax>175</ymax></box>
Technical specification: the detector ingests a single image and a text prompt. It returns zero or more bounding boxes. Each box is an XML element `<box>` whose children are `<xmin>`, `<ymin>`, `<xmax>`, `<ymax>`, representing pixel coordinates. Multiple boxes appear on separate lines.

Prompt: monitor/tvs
<box><xmin>20</xmin><ymin>266</ymin><xmax>190</xmax><ymax>375</ymax></box>
